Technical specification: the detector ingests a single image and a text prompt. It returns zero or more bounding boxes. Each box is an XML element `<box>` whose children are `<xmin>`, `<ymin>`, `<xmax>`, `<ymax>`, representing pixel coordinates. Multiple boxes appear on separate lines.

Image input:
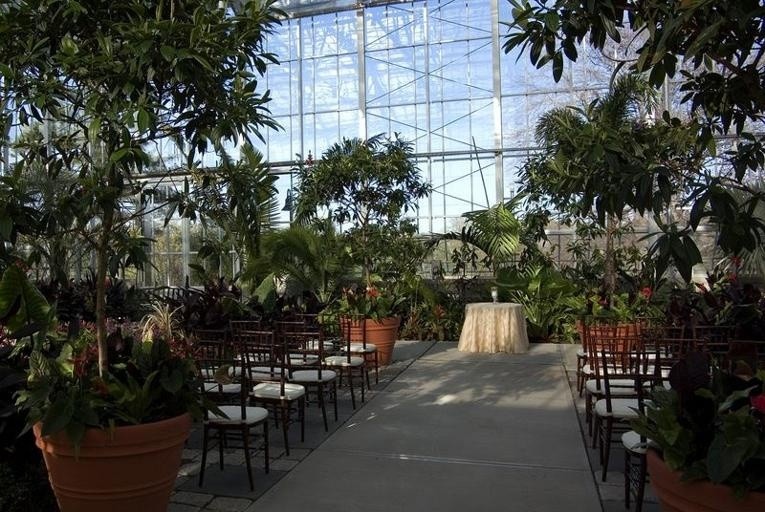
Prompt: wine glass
<box><xmin>490</xmin><ymin>286</ymin><xmax>498</xmax><ymax>304</ymax></box>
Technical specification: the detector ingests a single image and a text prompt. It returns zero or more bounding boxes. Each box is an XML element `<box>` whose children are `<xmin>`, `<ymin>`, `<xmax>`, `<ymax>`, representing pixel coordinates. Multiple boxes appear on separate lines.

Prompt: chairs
<box><xmin>189</xmin><ymin>310</ymin><xmax>379</xmax><ymax>492</ymax></box>
<box><xmin>575</xmin><ymin>315</ymin><xmax>765</xmax><ymax>512</ymax></box>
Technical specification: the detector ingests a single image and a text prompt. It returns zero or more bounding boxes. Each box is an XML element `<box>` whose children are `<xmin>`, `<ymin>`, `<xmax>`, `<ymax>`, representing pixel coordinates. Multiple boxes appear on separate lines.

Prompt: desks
<box><xmin>457</xmin><ymin>302</ymin><xmax>530</xmax><ymax>353</ymax></box>
